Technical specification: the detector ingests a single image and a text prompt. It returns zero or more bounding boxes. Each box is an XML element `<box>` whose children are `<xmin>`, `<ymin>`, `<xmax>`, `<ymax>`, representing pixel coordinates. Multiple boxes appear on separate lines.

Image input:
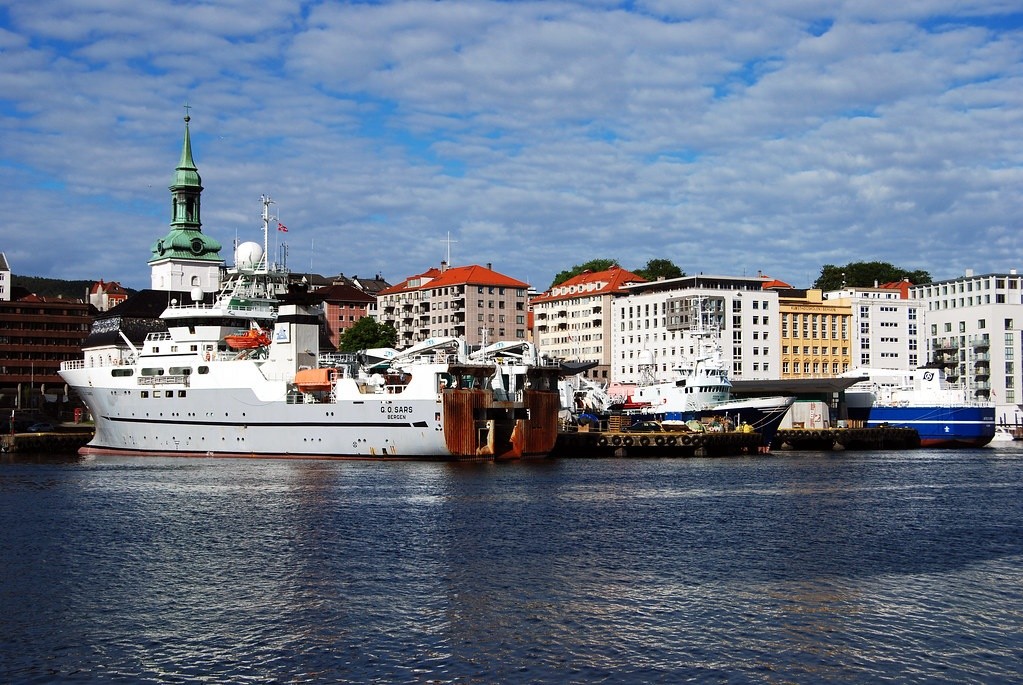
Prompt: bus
<box><xmin>0</xmin><ymin>407</ymin><xmax>42</xmax><ymax>415</ymax></box>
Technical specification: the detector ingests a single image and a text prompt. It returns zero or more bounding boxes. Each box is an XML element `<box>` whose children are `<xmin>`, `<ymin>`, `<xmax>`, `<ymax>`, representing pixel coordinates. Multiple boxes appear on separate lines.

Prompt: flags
<box><xmin>277</xmin><ymin>223</ymin><xmax>288</xmax><ymax>233</ymax></box>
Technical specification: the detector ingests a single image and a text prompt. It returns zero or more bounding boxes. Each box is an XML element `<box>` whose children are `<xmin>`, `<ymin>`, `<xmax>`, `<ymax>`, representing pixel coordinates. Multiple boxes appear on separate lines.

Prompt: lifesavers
<box><xmin>113</xmin><ymin>358</ymin><xmax>118</xmax><ymax>365</ymax></box>
<box><xmin>598</xmin><ymin>436</ymin><xmax>608</xmax><ymax>446</ymax></box>
<box><xmin>654</xmin><ymin>435</ymin><xmax>664</xmax><ymax>446</ymax></box>
<box><xmin>841</xmin><ymin>430</ymin><xmax>849</xmax><ymax>440</ymax></box>
<box><xmin>821</xmin><ymin>430</ymin><xmax>841</xmax><ymax>441</ymax></box>
<box><xmin>682</xmin><ymin>436</ymin><xmax>691</xmax><ymax>445</ymax></box>
<box><xmin>612</xmin><ymin>435</ymin><xmax>621</xmax><ymax>446</ymax></box>
<box><xmin>776</xmin><ymin>430</ymin><xmax>789</xmax><ymax>439</ymax></box>
<box><xmin>714</xmin><ymin>434</ymin><xmax>771</xmax><ymax>447</ymax></box>
<box><xmin>640</xmin><ymin>436</ymin><xmax>650</xmax><ymax>447</ymax></box>
<box><xmin>702</xmin><ymin>435</ymin><xmax>713</xmax><ymax>446</ymax></box>
<box><xmin>667</xmin><ymin>435</ymin><xmax>677</xmax><ymax>446</ymax></box>
<box><xmin>206</xmin><ymin>352</ymin><xmax>211</xmax><ymax>362</ymax></box>
<box><xmin>790</xmin><ymin>431</ymin><xmax>812</xmax><ymax>440</ymax></box>
<box><xmin>812</xmin><ymin>431</ymin><xmax>819</xmax><ymax>440</ymax></box>
<box><xmin>692</xmin><ymin>435</ymin><xmax>702</xmax><ymax>446</ymax></box>
<box><xmin>849</xmin><ymin>430</ymin><xmax>918</xmax><ymax>441</ymax></box>
<box><xmin>624</xmin><ymin>436</ymin><xmax>633</xmax><ymax>446</ymax></box>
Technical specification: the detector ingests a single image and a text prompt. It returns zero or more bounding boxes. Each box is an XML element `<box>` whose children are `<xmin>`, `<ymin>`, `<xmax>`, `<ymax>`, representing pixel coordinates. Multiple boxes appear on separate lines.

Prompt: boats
<box><xmin>836</xmin><ymin>352</ymin><xmax>996</xmax><ymax>448</ymax></box>
<box><xmin>557</xmin><ymin>294</ymin><xmax>797</xmax><ymax>456</ymax></box>
<box><xmin>56</xmin><ymin>193</ymin><xmax>599</xmax><ymax>460</ymax></box>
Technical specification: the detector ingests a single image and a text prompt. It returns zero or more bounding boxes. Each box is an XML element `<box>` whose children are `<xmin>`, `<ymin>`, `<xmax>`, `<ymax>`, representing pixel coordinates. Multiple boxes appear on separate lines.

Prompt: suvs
<box><xmin>27</xmin><ymin>422</ymin><xmax>54</xmax><ymax>432</ymax></box>
